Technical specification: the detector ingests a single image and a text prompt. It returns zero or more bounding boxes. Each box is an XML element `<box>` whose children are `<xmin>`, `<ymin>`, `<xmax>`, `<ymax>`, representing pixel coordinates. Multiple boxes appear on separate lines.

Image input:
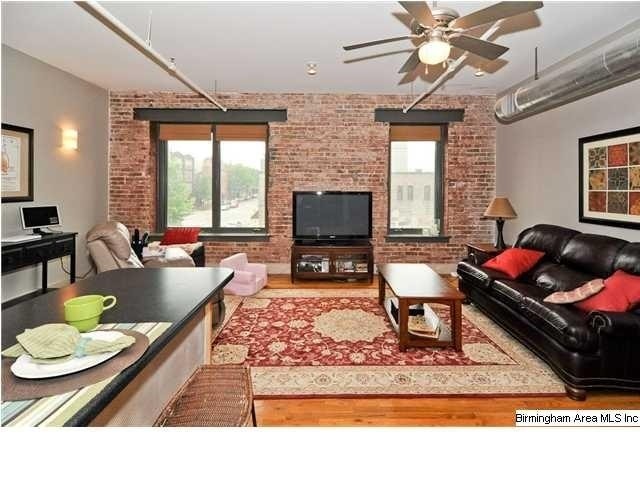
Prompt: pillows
<box><xmin>571</xmin><ymin>268</ymin><xmax>640</xmax><ymax>318</ymax></box>
<box><xmin>542</xmin><ymin>278</ymin><xmax>605</xmax><ymax>303</ymax></box>
<box><xmin>479</xmin><ymin>246</ymin><xmax>547</xmax><ymax>280</ymax></box>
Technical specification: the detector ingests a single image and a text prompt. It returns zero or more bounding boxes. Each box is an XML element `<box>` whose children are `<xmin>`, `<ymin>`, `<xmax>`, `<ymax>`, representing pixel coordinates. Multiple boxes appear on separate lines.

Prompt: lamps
<box><xmin>417</xmin><ymin>31</ymin><xmax>451</xmax><ymax>64</ymax></box>
<box><xmin>58</xmin><ymin>127</ymin><xmax>81</xmax><ymax>152</ymax></box>
<box><xmin>484</xmin><ymin>197</ymin><xmax>517</xmax><ymax>249</ymax></box>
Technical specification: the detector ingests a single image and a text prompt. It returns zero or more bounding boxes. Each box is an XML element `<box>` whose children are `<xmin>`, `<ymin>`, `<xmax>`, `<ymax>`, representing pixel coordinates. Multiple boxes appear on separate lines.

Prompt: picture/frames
<box><xmin>578</xmin><ymin>126</ymin><xmax>640</xmax><ymax>230</ymax></box>
<box><xmin>1</xmin><ymin>122</ymin><xmax>34</xmax><ymax>203</ymax></box>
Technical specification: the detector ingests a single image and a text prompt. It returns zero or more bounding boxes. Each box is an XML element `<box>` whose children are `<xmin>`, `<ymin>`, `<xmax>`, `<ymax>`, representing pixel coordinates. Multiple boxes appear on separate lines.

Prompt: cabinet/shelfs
<box><xmin>148</xmin><ymin>240</ymin><xmax>205</xmax><ymax>268</ymax></box>
<box><xmin>290</xmin><ymin>241</ymin><xmax>373</xmax><ymax>285</ymax></box>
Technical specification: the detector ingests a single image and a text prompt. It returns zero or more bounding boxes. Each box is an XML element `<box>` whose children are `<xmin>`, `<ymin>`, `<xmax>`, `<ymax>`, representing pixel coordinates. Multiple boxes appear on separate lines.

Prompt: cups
<box><xmin>321</xmin><ymin>260</ymin><xmax>329</xmax><ymax>274</ymax></box>
<box><xmin>63</xmin><ymin>293</ymin><xmax>117</xmax><ymax>333</ymax></box>
<box><xmin>422</xmin><ymin>218</ymin><xmax>441</xmax><ymax>236</ymax></box>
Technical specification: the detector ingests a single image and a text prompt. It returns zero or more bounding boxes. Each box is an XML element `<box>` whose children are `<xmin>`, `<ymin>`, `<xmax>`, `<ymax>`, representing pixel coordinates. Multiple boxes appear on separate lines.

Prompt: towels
<box><xmin>0</xmin><ymin>318</ymin><xmax>139</xmax><ymax>365</ymax></box>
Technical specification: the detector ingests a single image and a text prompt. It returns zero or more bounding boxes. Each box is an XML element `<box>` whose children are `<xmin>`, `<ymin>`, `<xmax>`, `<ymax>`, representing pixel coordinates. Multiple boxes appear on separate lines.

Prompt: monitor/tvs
<box><xmin>20</xmin><ymin>204</ymin><xmax>62</xmax><ymax>236</ymax></box>
<box><xmin>292</xmin><ymin>191</ymin><xmax>372</xmax><ymax>246</ymax></box>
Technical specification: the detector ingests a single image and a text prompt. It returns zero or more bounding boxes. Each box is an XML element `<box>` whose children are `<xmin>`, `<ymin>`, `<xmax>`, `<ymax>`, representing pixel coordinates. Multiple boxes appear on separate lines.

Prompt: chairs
<box><xmin>86</xmin><ymin>220</ymin><xmax>226</xmax><ymax>329</ymax></box>
<box><xmin>220</xmin><ymin>253</ymin><xmax>268</xmax><ymax>297</ymax></box>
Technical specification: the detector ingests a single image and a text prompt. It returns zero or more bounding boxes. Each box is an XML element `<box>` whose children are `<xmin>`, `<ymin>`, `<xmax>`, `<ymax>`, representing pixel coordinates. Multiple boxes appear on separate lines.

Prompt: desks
<box><xmin>1</xmin><ymin>266</ymin><xmax>236</xmax><ymax>427</ymax></box>
<box><xmin>1</xmin><ymin>231</ymin><xmax>79</xmax><ymax>311</ymax></box>
<box><xmin>466</xmin><ymin>242</ymin><xmax>510</xmax><ymax>263</ymax></box>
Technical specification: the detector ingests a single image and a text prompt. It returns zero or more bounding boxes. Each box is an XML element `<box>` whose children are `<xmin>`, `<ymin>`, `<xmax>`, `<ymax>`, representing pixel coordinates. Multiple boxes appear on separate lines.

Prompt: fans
<box><xmin>342</xmin><ymin>1</ymin><xmax>545</xmax><ymax>74</ymax></box>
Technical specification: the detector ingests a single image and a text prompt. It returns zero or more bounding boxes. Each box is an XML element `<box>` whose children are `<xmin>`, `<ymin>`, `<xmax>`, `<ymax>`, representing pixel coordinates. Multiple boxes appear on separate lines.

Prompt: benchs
<box><xmin>151</xmin><ymin>362</ymin><xmax>258</xmax><ymax>427</ymax></box>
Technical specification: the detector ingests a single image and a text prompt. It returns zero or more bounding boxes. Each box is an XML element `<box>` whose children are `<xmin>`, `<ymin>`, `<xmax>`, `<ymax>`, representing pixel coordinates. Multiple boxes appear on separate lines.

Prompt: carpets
<box><xmin>211</xmin><ymin>287</ymin><xmax>571</xmax><ymax>399</ymax></box>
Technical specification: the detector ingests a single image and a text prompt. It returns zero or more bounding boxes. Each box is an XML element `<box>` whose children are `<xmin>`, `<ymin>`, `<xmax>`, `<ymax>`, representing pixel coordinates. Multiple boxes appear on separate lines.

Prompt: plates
<box><xmin>11</xmin><ymin>330</ymin><xmax>130</xmax><ymax>379</ymax></box>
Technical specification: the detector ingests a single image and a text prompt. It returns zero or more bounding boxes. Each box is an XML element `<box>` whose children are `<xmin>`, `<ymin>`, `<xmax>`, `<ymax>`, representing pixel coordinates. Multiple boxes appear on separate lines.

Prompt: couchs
<box><xmin>457</xmin><ymin>223</ymin><xmax>639</xmax><ymax>401</ymax></box>
